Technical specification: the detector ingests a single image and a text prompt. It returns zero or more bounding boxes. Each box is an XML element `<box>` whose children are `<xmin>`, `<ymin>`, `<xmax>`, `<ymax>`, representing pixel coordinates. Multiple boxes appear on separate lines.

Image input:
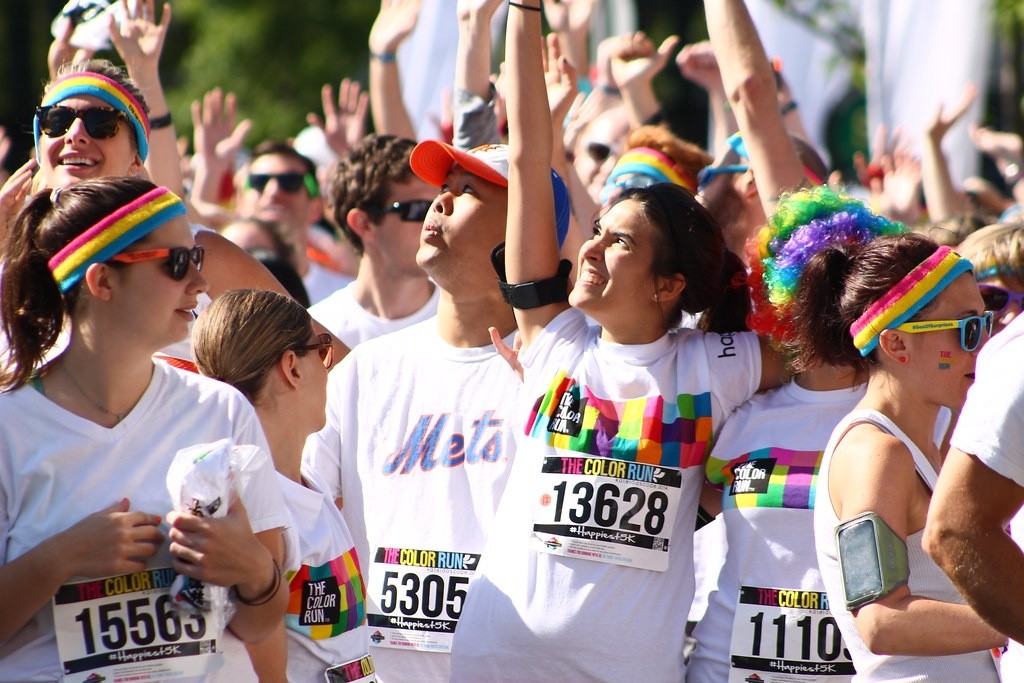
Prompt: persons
<box><xmin>0</xmin><ymin>0</ymin><xmax>1024</xmax><ymax>683</ymax></box>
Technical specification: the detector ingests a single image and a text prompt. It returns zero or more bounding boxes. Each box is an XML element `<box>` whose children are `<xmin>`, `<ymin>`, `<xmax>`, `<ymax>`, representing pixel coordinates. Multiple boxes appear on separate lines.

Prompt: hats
<box><xmin>410</xmin><ymin>140</ymin><xmax>570</xmax><ymax>250</ymax></box>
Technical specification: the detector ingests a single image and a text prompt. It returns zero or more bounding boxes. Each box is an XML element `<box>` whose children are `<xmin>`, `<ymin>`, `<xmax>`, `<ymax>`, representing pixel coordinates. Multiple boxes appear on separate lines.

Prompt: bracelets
<box><xmin>647</xmin><ymin>109</ymin><xmax>663</xmax><ymax>125</ymax></box>
<box><xmin>596</xmin><ymin>83</ymin><xmax>618</xmax><ymax>94</ymax></box>
<box><xmin>509</xmin><ymin>2</ymin><xmax>540</xmax><ymax>11</ymax></box>
<box><xmin>235</xmin><ymin>560</ymin><xmax>281</xmax><ymax>605</ymax></box>
<box><xmin>369</xmin><ymin>51</ymin><xmax>395</xmax><ymax>62</ymax></box>
<box><xmin>150</xmin><ymin>114</ymin><xmax>171</xmax><ymax>128</ymax></box>
<box><xmin>781</xmin><ymin>101</ymin><xmax>797</xmax><ymax>114</ymax></box>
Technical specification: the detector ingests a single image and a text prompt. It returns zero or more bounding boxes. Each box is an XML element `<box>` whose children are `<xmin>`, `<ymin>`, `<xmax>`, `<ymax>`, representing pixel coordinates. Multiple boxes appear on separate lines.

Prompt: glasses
<box><xmin>697</xmin><ymin>165</ymin><xmax>748</xmax><ymax>191</ymax></box>
<box><xmin>110</xmin><ymin>246</ymin><xmax>205</xmax><ymax>281</ymax></box>
<box><xmin>896</xmin><ymin>310</ymin><xmax>995</xmax><ymax>351</ymax></box>
<box><xmin>359</xmin><ymin>200</ymin><xmax>432</xmax><ymax>221</ymax></box>
<box><xmin>250</xmin><ymin>173</ymin><xmax>304</xmax><ymax>193</ymax></box>
<box><xmin>293</xmin><ymin>333</ymin><xmax>333</xmax><ymax>368</ymax></box>
<box><xmin>35</xmin><ymin>105</ymin><xmax>125</xmax><ymax>140</ymax></box>
<box><xmin>587</xmin><ymin>143</ymin><xmax>616</xmax><ymax>163</ymax></box>
<box><xmin>977</xmin><ymin>284</ymin><xmax>1024</xmax><ymax>316</ymax></box>
<box><xmin>600</xmin><ymin>175</ymin><xmax>657</xmax><ymax>204</ymax></box>
